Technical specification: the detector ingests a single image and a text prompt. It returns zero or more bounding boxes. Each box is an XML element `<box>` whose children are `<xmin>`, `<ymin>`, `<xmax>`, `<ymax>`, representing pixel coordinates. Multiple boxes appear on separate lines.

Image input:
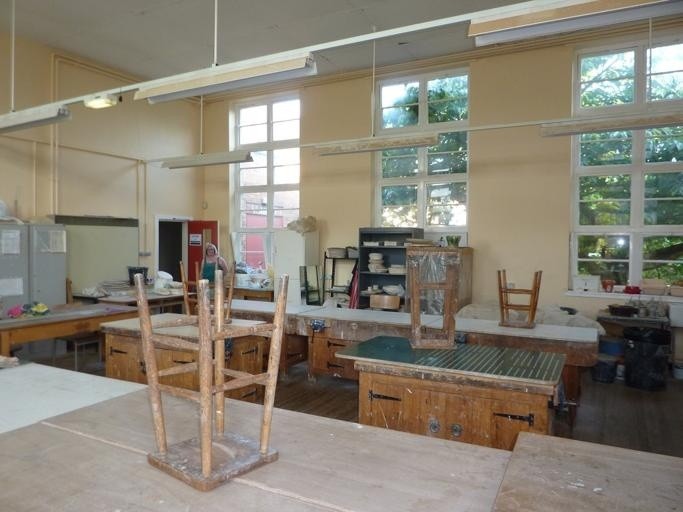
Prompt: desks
<box><xmin>0</xmin><ymin>289</ymin><xmax>197</xmax><ymax>358</ymax></box>
<box><xmin>1</xmin><ymin>363</ymin><xmax>511</xmax><ymax>511</ymax></box>
<box><xmin>594</xmin><ymin>312</ymin><xmax>674</xmax><ymax>382</ymax></box>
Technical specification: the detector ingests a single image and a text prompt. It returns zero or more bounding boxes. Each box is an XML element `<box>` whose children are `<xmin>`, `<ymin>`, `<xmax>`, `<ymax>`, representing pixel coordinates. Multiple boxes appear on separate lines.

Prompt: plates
<box><xmin>405</xmin><ymin>238</ymin><xmax>433</xmax><ymax>243</ymax></box>
<box><xmin>375</xmin><ymin>269</ymin><xmax>387</xmax><ymax>273</ymax></box>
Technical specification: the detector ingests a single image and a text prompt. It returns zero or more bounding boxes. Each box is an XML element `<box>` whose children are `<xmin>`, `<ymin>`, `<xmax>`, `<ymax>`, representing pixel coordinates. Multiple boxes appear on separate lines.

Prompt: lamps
<box><xmin>82</xmin><ymin>90</ymin><xmax>122</xmax><ymax>111</ymax></box>
<box><xmin>536</xmin><ymin>104</ymin><xmax>682</xmax><ymax>142</ymax></box>
<box><xmin>464</xmin><ymin>1</ymin><xmax>682</xmax><ymax>50</ymax></box>
<box><xmin>131</xmin><ymin>49</ymin><xmax>324</xmax><ymax>108</ymax></box>
<box><xmin>312</xmin><ymin>129</ymin><xmax>445</xmax><ymax>161</ymax></box>
<box><xmin>156</xmin><ymin>147</ymin><xmax>259</xmax><ymax>173</ymax></box>
<box><xmin>0</xmin><ymin>102</ymin><xmax>73</xmax><ymax>138</ymax></box>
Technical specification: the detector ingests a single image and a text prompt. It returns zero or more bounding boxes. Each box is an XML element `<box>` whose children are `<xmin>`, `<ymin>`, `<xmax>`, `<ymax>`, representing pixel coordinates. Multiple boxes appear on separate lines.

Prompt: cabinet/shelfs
<box><xmin>321</xmin><ymin>226</ymin><xmax>424</xmax><ymax>315</ymax></box>
<box><xmin>306</xmin><ymin>316</ymin><xmax>569</xmax><ymax>457</ymax></box>
<box><xmin>100</xmin><ymin>325</ymin><xmax>266</xmax><ymax>407</ymax></box>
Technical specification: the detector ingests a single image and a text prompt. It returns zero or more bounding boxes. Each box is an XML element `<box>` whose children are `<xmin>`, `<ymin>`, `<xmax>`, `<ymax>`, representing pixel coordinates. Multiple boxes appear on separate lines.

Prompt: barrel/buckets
<box><xmin>128</xmin><ymin>265</ymin><xmax>148</xmax><ymax>285</ymax></box>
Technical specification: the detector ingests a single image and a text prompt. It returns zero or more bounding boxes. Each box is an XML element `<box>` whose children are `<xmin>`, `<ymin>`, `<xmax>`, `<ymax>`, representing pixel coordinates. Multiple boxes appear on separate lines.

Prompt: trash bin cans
<box><xmin>623</xmin><ymin>327</ymin><xmax>671</xmax><ymax>391</ymax></box>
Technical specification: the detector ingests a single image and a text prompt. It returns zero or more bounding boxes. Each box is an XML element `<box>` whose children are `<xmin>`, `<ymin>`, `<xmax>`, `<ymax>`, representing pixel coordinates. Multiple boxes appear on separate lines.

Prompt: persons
<box><xmin>198</xmin><ymin>243</ymin><xmax>227</xmax><ymax>299</ymax></box>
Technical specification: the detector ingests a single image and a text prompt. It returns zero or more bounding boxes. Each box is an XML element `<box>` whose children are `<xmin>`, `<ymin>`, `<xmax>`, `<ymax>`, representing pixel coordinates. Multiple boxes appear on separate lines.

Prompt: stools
<box><xmin>53</xmin><ymin>335</ymin><xmax>103</xmax><ymax>371</ymax></box>
<box><xmin>405</xmin><ymin>256</ymin><xmax>544</xmax><ymax>350</ymax></box>
<box><xmin>130</xmin><ymin>256</ymin><xmax>290</xmax><ymax>490</ymax></box>
